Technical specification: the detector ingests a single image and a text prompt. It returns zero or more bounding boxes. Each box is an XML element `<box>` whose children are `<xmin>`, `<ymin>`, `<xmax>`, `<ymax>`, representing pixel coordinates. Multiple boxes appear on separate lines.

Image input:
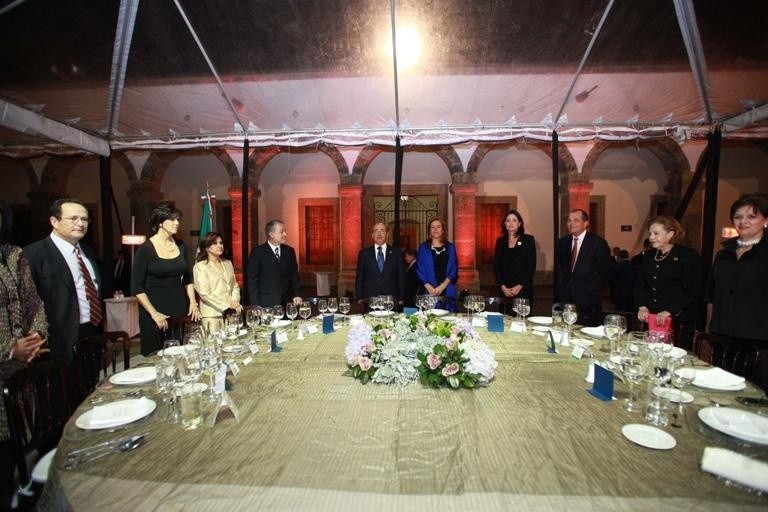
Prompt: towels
<box><xmin>701</xmin><ymin>444</ymin><xmax>768</xmax><ymax>493</ymax></box>
<box><xmin>683</xmin><ymin>367</ymin><xmax>745</xmax><ymax>390</ymax></box>
<box><xmin>89</xmin><ymin>397</ymin><xmax>146</xmax><ymax>425</ymax></box>
<box><xmin>713</xmin><ymin>407</ymin><xmax>768</xmax><ymax>440</ymax></box>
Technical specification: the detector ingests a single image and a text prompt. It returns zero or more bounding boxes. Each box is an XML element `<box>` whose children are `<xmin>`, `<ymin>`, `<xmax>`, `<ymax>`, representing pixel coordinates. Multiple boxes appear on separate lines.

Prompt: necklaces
<box><xmin>736</xmin><ymin>236</ymin><xmax>760</xmax><ymax>248</ymax></box>
<box><xmin>654</xmin><ymin>247</ymin><xmax>674</xmax><ymax>261</ymax></box>
<box><xmin>431</xmin><ymin>245</ymin><xmax>447</xmax><ymax>256</ymax></box>
<box><xmin>154</xmin><ymin>235</ymin><xmax>177</xmax><ymax>253</ymax></box>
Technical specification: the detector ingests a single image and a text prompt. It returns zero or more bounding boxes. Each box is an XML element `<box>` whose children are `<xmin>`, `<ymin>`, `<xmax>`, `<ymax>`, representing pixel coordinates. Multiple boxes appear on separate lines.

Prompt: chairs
<box><xmin>157</xmin><ymin>319</ymin><xmax>186</xmax><ymax>345</ymax></box>
<box><xmin>291</xmin><ymin>297</ymin><xmax>326</xmax><ymax>318</ymax></box>
<box><xmin>485</xmin><ymin>295</ymin><xmax>532</xmax><ymax>320</ymax></box>
<box><xmin>3</xmin><ymin>368</ymin><xmax>67</xmax><ymax>485</ymax></box>
<box><xmin>358</xmin><ymin>298</ymin><xmax>403</xmax><ymax>314</ymax></box>
<box><xmin>603</xmin><ymin>309</ymin><xmax>643</xmax><ymax>336</ymax></box>
<box><xmin>220</xmin><ymin>305</ymin><xmax>264</xmax><ymax>327</ymax></box>
<box><xmin>693</xmin><ymin>328</ymin><xmax>759</xmax><ymax>377</ymax></box>
<box><xmin>73</xmin><ymin>332</ymin><xmax>133</xmax><ymax>387</ymax></box>
<box><xmin>414</xmin><ymin>295</ymin><xmax>457</xmax><ymax>311</ymax></box>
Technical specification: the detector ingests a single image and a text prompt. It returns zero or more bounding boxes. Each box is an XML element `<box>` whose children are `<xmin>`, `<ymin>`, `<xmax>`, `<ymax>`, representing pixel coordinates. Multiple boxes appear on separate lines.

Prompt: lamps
<box><xmin>576</xmin><ymin>85</ymin><xmax>603</xmax><ymax>102</ymax></box>
<box><xmin>231</xmin><ymin>98</ymin><xmax>244</xmax><ymax>109</ymax></box>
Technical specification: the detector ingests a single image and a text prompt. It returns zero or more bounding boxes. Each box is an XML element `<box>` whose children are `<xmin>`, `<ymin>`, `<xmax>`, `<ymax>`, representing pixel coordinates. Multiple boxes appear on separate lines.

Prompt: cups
<box><xmin>618</xmin><ymin>353</ymin><xmax>649</xmax><ymax>414</ymax></box>
<box><xmin>369</xmin><ymin>296</ymin><xmax>379</xmax><ymax>323</ymax></box>
<box><xmin>182</xmin><ymin>333</ymin><xmax>204</xmax><ymax>346</ymax></box>
<box><xmin>384</xmin><ymin>296</ymin><xmax>395</xmax><ymax>326</ymax></box>
<box><xmin>518</xmin><ymin>298</ymin><xmax>531</xmax><ymax>324</ymax></box>
<box><xmin>512</xmin><ymin>298</ymin><xmax>520</xmax><ymax>321</ymax></box>
<box><xmin>473</xmin><ymin>296</ymin><xmax>486</xmax><ymax>317</ymax></box>
<box><xmin>339</xmin><ymin>297</ymin><xmax>351</xmax><ymax>328</ymax></box>
<box><xmin>246</xmin><ymin>310</ymin><xmax>261</xmax><ymax>342</ymax></box>
<box><xmin>299</xmin><ymin>301</ymin><xmax>311</xmax><ymax>326</ymax></box>
<box><xmin>286</xmin><ymin>303</ymin><xmax>298</xmax><ymax>334</ymax></box>
<box><xmin>180</xmin><ymin>388</ymin><xmax>204</xmax><ymax>429</ymax></box>
<box><xmin>604</xmin><ymin>316</ymin><xmax>622</xmax><ymax>357</ymax></box>
<box><xmin>415</xmin><ymin>295</ymin><xmax>424</xmax><ymax>318</ymax></box>
<box><xmin>199</xmin><ymin>349</ymin><xmax>222</xmax><ymax>402</ymax></box>
<box><xmin>208</xmin><ymin>319</ymin><xmax>222</xmax><ymax>332</ymax></box>
<box><xmin>190</xmin><ymin>324</ymin><xmax>207</xmax><ymax>339</ymax></box>
<box><xmin>671</xmin><ymin>358</ymin><xmax>697</xmax><ymax>412</ymax></box>
<box><xmin>627</xmin><ymin>331</ymin><xmax>649</xmax><ymax>357</ymax></box>
<box><xmin>655</xmin><ymin>331</ymin><xmax>673</xmax><ymax>362</ymax></box>
<box><xmin>327</xmin><ymin>298</ymin><xmax>338</xmax><ymax>324</ymax></box>
<box><xmin>377</xmin><ymin>296</ymin><xmax>387</xmax><ymax>325</ymax></box>
<box><xmin>649</xmin><ymin>355</ymin><xmax>674</xmax><ymax>410</ymax></box>
<box><xmin>272</xmin><ymin>304</ymin><xmax>285</xmax><ymax>327</ymax></box>
<box><xmin>468</xmin><ymin>296</ymin><xmax>475</xmax><ymax>321</ymax></box>
<box><xmin>260</xmin><ymin>307</ymin><xmax>273</xmax><ymax>331</ymax></box>
<box><xmin>425</xmin><ymin>296</ymin><xmax>435</xmax><ymax>318</ymax></box>
<box><xmin>177</xmin><ymin>355</ymin><xmax>203</xmax><ymax>388</ymax></box>
<box><xmin>463</xmin><ymin>296</ymin><xmax>468</xmax><ymax>322</ymax></box>
<box><xmin>229</xmin><ymin>313</ymin><xmax>243</xmax><ymax>348</ymax></box>
<box><xmin>318</xmin><ymin>300</ymin><xmax>328</xmax><ymax>318</ymax></box>
<box><xmin>224</xmin><ymin>318</ymin><xmax>241</xmax><ymax>358</ymax></box>
<box><xmin>616</xmin><ymin>317</ymin><xmax>627</xmax><ymax>350</ymax></box>
<box><xmin>562</xmin><ymin>304</ymin><xmax>578</xmax><ymax>340</ymax></box>
<box><xmin>159</xmin><ymin>354</ymin><xmax>177</xmax><ymax>391</ymax></box>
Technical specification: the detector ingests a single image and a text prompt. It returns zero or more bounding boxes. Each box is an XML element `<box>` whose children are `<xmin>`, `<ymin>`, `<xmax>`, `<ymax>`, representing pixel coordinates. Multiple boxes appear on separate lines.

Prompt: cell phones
<box><xmin>735</xmin><ymin>396</ymin><xmax>768</xmax><ymax>407</ymax></box>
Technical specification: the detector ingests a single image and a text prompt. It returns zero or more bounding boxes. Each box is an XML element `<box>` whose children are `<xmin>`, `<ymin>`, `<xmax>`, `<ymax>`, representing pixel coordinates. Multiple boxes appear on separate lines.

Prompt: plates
<box><xmin>698</xmin><ymin>406</ymin><xmax>768</xmax><ymax>446</ymax></box>
<box><xmin>652</xmin><ymin>386</ymin><xmax>695</xmax><ymax>404</ymax></box>
<box><xmin>108</xmin><ymin>366</ymin><xmax>159</xmax><ymax>387</ymax></box>
<box><xmin>411</xmin><ymin>308</ymin><xmax>450</xmax><ymax>317</ymax></box>
<box><xmin>581</xmin><ymin>327</ymin><xmax>604</xmax><ymax>338</ymax></box>
<box><xmin>673</xmin><ymin>367</ymin><xmax>747</xmax><ymax>392</ymax></box>
<box><xmin>75</xmin><ymin>397</ymin><xmax>157</xmax><ymax>431</ymax></box>
<box><xmin>630</xmin><ymin>342</ymin><xmax>688</xmax><ymax>361</ymax></box>
<box><xmin>527</xmin><ymin>316</ymin><xmax>554</xmax><ymax>326</ymax></box>
<box><xmin>223</xmin><ymin>345</ymin><xmax>243</xmax><ymax>354</ymax></box>
<box><xmin>368</xmin><ymin>310</ymin><xmax>396</xmax><ymax>317</ymax></box>
<box><xmin>621</xmin><ymin>422</ymin><xmax>677</xmax><ymax>450</ymax></box>
<box><xmin>268</xmin><ymin>319</ymin><xmax>292</xmax><ymax>329</ymax></box>
<box><xmin>156</xmin><ymin>345</ymin><xmax>199</xmax><ymax>358</ymax></box>
<box><xmin>216</xmin><ymin>328</ymin><xmax>248</xmax><ymax>339</ymax></box>
<box><xmin>473</xmin><ymin>311</ymin><xmax>503</xmax><ymax>318</ymax></box>
<box><xmin>317</xmin><ymin>313</ymin><xmax>349</xmax><ymax>321</ymax></box>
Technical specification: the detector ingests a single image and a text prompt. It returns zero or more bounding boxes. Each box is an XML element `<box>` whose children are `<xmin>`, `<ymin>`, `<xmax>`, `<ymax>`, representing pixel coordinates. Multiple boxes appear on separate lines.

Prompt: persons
<box><xmin>555</xmin><ymin>209</ymin><xmax>611</xmax><ymax>326</ymax></box>
<box><xmin>130</xmin><ymin>204</ymin><xmax>202</xmax><ymax>356</ymax></box>
<box><xmin>405</xmin><ymin>249</ymin><xmax>418</xmax><ymax>307</ymax></box>
<box><xmin>246</xmin><ymin>220</ymin><xmax>302</xmax><ymax>312</ymax></box>
<box><xmin>631</xmin><ymin>236</ymin><xmax>652</xmax><ymax>278</ymax></box>
<box><xmin>705</xmin><ymin>196</ymin><xmax>767</xmax><ymax>393</ymax></box>
<box><xmin>493</xmin><ymin>210</ymin><xmax>537</xmax><ymax>316</ymax></box>
<box><xmin>22</xmin><ymin>199</ymin><xmax>107</xmax><ymax>419</ymax></box>
<box><xmin>631</xmin><ymin>216</ymin><xmax>703</xmax><ymax>348</ymax></box>
<box><xmin>193</xmin><ymin>233</ymin><xmax>243</xmax><ymax>335</ymax></box>
<box><xmin>610</xmin><ymin>246</ymin><xmax>620</xmax><ymax>264</ymax></box>
<box><xmin>0</xmin><ymin>198</ymin><xmax>49</xmax><ymax>512</ymax></box>
<box><xmin>355</xmin><ymin>223</ymin><xmax>407</xmax><ymax>312</ymax></box>
<box><xmin>417</xmin><ymin>217</ymin><xmax>459</xmax><ymax>311</ymax></box>
<box><xmin>611</xmin><ymin>250</ymin><xmax>633</xmax><ymax>313</ymax></box>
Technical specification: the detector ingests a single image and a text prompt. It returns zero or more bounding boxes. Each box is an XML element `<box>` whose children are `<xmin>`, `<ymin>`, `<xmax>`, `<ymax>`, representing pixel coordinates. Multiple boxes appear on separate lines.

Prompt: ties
<box><xmin>75</xmin><ymin>247</ymin><xmax>104</xmax><ymax>326</ymax></box>
<box><xmin>569</xmin><ymin>238</ymin><xmax>578</xmax><ymax>272</ymax></box>
<box><xmin>377</xmin><ymin>246</ymin><xmax>385</xmax><ymax>273</ymax></box>
<box><xmin>275</xmin><ymin>248</ymin><xmax>281</xmax><ymax>264</ymax></box>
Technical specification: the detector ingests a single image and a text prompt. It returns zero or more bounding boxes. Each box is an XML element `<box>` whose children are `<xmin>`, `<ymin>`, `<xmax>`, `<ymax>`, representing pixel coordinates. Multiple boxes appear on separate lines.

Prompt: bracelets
<box><xmin>152</xmin><ymin>312</ymin><xmax>160</xmax><ymax>319</ymax></box>
<box><xmin>190</xmin><ymin>303</ymin><xmax>198</xmax><ymax>306</ymax></box>
<box><xmin>6</xmin><ymin>346</ymin><xmax>17</xmax><ymax>362</ymax></box>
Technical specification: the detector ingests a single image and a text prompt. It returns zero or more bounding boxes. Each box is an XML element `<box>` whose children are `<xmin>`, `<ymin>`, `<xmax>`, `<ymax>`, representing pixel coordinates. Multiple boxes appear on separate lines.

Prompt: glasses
<box><xmin>59</xmin><ymin>217</ymin><xmax>91</xmax><ymax>224</ymax></box>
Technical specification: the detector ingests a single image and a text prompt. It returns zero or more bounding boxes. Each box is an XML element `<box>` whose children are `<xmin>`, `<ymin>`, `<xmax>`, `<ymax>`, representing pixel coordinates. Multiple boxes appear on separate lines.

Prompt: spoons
<box><xmin>64</xmin><ymin>434</ymin><xmax>147</xmax><ymax>473</ymax></box>
